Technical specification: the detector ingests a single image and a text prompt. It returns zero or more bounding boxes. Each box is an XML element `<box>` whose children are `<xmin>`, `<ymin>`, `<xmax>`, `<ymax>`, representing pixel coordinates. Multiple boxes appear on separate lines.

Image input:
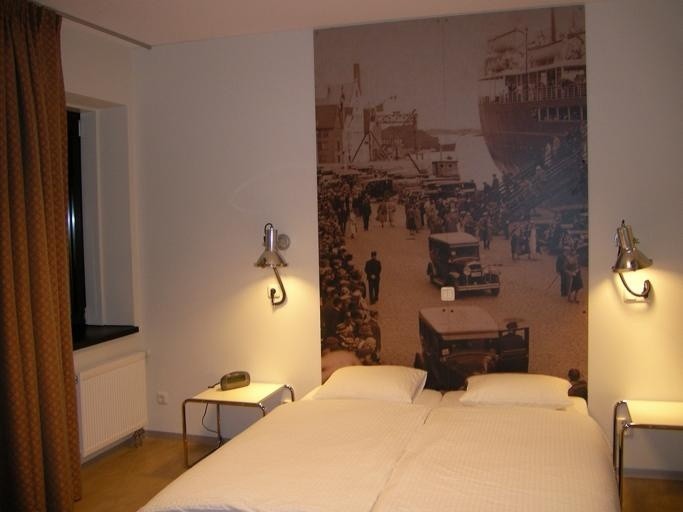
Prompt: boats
<box><xmin>473</xmin><ymin>9</ymin><xmax>586</xmax><ymax>177</ymax></box>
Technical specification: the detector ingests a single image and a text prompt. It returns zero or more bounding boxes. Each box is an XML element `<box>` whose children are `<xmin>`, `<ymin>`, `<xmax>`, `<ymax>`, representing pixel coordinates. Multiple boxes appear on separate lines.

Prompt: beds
<box><xmin>135</xmin><ymin>384</ymin><xmax>615</xmax><ymax>511</ymax></box>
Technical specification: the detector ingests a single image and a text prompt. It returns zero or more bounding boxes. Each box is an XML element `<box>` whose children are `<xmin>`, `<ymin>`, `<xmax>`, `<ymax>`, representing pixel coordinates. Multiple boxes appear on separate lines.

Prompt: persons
<box><xmin>567</xmin><ymin>367</ymin><xmax>587</xmax><ymax>402</ymax></box>
<box><xmin>501</xmin><ymin>322</ymin><xmax>523</xmax><ymax>349</ymax></box>
<box><xmin>317</xmin><ymin>175</ymin><xmax>382</xmax><ymax>384</ymax></box>
<box><xmin>381</xmin><ymin>180</ymin><xmax>588</xmax><ymax>302</ymax></box>
<box><xmin>499</xmin><ymin>73</ymin><xmax>587</xmax><ymax>180</ymax></box>
<box><xmin>482</xmin><ymin>347</ymin><xmax>501</xmax><ymax>373</ymax></box>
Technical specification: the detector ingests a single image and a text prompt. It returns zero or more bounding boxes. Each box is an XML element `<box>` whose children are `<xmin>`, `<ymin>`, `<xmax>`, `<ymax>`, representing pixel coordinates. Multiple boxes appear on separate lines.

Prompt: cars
<box><xmin>413</xmin><ymin>304</ymin><xmax>531</xmax><ymax>393</ymax></box>
<box><xmin>426</xmin><ymin>232</ymin><xmax>500</xmax><ymax>297</ymax></box>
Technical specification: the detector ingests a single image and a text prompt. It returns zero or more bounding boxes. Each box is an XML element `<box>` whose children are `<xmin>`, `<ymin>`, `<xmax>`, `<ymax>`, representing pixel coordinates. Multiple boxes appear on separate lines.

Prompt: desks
<box><xmin>182</xmin><ymin>384</ymin><xmax>294</xmax><ymax>466</ymax></box>
<box><xmin>613</xmin><ymin>400</ymin><xmax>683</xmax><ymax>499</ymax></box>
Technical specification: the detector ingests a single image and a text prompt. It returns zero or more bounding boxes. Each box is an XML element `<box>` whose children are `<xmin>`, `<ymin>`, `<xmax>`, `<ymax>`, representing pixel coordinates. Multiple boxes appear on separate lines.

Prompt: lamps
<box><xmin>611</xmin><ymin>220</ymin><xmax>654</xmax><ymax>300</ymax></box>
<box><xmin>254</xmin><ymin>222</ymin><xmax>289</xmax><ymax>307</ymax></box>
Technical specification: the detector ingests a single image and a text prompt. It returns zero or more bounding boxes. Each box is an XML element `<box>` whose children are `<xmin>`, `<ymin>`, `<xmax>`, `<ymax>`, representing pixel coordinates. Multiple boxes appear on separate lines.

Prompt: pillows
<box><xmin>309</xmin><ymin>365</ymin><xmax>570</xmax><ymax>409</ymax></box>
